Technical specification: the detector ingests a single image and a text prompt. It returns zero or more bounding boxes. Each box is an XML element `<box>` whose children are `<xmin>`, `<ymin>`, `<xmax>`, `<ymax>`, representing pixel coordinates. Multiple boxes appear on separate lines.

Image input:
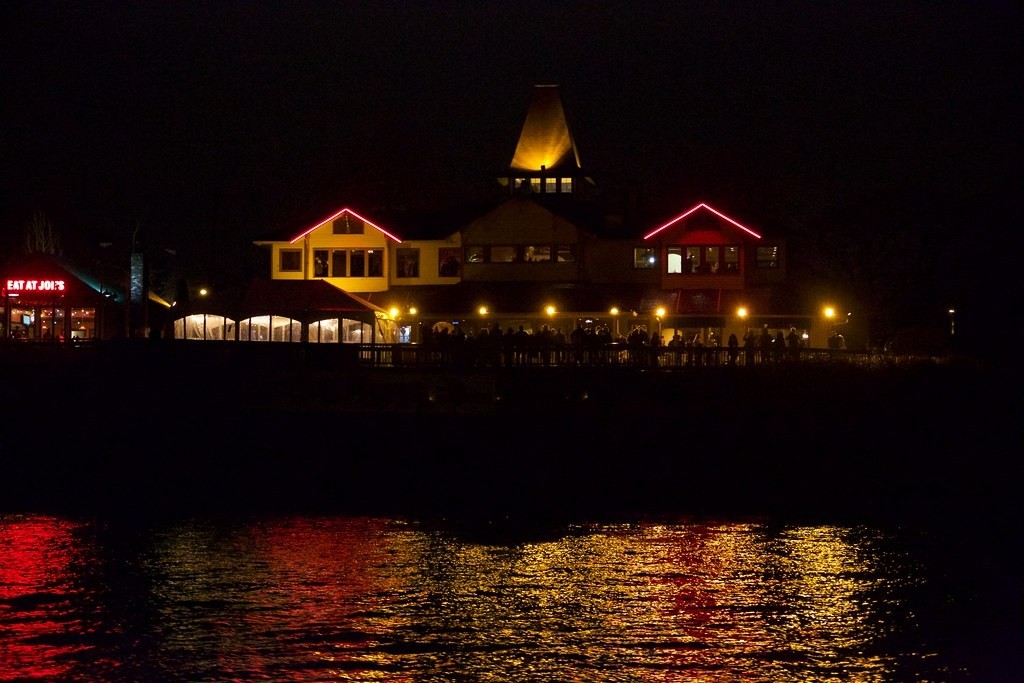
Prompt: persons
<box><xmin>628</xmin><ymin>330</ymin><xmax>660</xmax><ymax>365</ymax></box>
<box><xmin>728</xmin><ymin>334</ymin><xmax>738</xmax><ymax>366</ymax></box>
<box><xmin>743</xmin><ymin>332</ymin><xmax>755</xmax><ymax>367</ymax></box>
<box><xmin>787</xmin><ymin>328</ymin><xmax>799</xmax><ymax>356</ymax></box>
<box><xmin>669</xmin><ymin>337</ymin><xmax>684</xmax><ymax>368</ymax></box>
<box><xmin>423</xmin><ymin>322</ymin><xmax>565</xmax><ymax>366</ymax></box>
<box><xmin>570</xmin><ymin>326</ymin><xmax>612</xmax><ymax>364</ymax></box>
<box><xmin>440</xmin><ymin>257</ymin><xmax>459</xmax><ymax>277</ymax></box>
<box><xmin>693</xmin><ymin>335</ymin><xmax>701</xmax><ymax>367</ymax></box>
<box><xmin>687</xmin><ymin>253</ymin><xmax>700</xmax><ymax>273</ymax></box>
<box><xmin>759</xmin><ymin>329</ymin><xmax>786</xmax><ymax>367</ymax></box>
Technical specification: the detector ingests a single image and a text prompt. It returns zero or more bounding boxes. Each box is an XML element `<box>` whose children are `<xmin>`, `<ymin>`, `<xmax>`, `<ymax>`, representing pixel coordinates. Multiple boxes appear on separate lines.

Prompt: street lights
<box><xmin>408</xmin><ymin>307</ymin><xmax>419</xmax><ymax>345</ymax></box>
<box><xmin>610</xmin><ymin>308</ymin><xmax>618</xmax><ymax>344</ymax></box>
<box><xmin>738</xmin><ymin>309</ymin><xmax>746</xmax><ymax>347</ymax></box>
<box><xmin>546</xmin><ymin>306</ymin><xmax>555</xmax><ymax>344</ymax></box>
<box><xmin>824</xmin><ymin>308</ymin><xmax>833</xmax><ymax>349</ymax></box>
<box><xmin>656</xmin><ymin>308</ymin><xmax>665</xmax><ymax>347</ymax></box>
